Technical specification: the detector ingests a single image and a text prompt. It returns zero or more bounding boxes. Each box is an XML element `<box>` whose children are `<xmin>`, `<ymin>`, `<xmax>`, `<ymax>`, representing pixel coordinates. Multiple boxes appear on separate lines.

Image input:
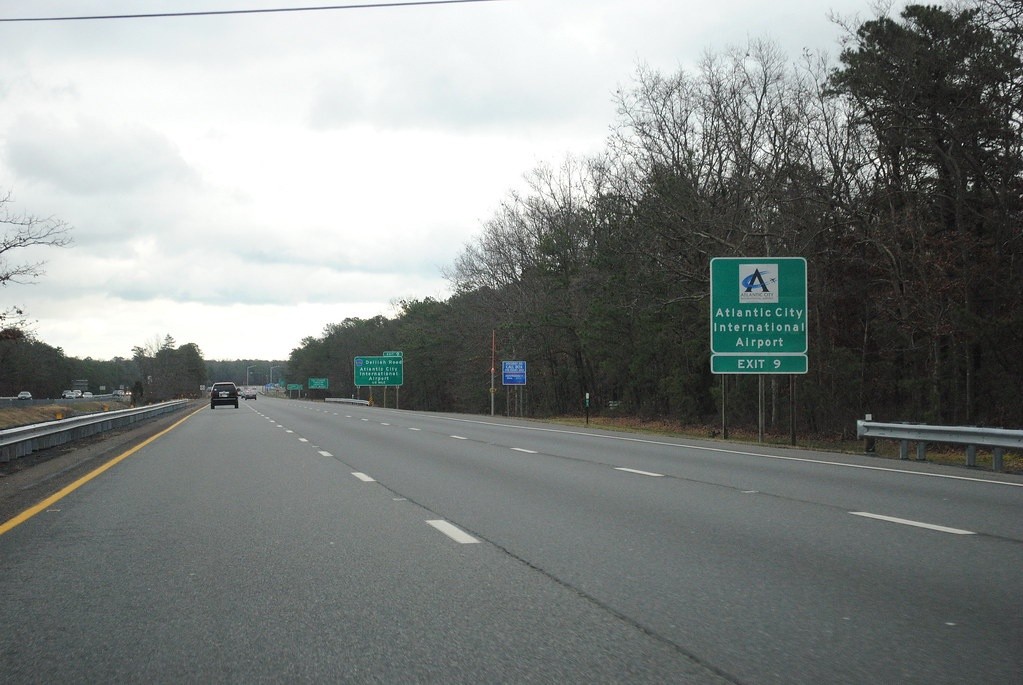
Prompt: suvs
<box><xmin>207</xmin><ymin>382</ymin><xmax>241</xmax><ymax>409</ymax></box>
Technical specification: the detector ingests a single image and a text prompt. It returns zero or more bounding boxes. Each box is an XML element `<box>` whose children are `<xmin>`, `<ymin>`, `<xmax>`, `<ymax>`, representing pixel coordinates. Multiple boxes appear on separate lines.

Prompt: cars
<box><xmin>241</xmin><ymin>388</ymin><xmax>249</xmax><ymax>399</ymax></box>
<box><xmin>237</xmin><ymin>390</ymin><xmax>243</xmax><ymax>395</ymax></box>
<box><xmin>245</xmin><ymin>389</ymin><xmax>256</xmax><ymax>400</ymax></box>
<box><xmin>73</xmin><ymin>390</ymin><xmax>82</xmax><ymax>398</ymax></box>
<box><xmin>18</xmin><ymin>392</ymin><xmax>32</xmax><ymax>399</ymax></box>
<box><xmin>62</xmin><ymin>390</ymin><xmax>71</xmax><ymax>399</ymax></box>
<box><xmin>113</xmin><ymin>389</ymin><xmax>132</xmax><ymax>397</ymax></box>
<box><xmin>83</xmin><ymin>392</ymin><xmax>94</xmax><ymax>399</ymax></box>
<box><xmin>66</xmin><ymin>392</ymin><xmax>76</xmax><ymax>399</ymax></box>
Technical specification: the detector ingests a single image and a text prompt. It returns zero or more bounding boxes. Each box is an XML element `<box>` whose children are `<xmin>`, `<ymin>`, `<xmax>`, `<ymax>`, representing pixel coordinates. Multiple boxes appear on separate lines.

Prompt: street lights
<box><xmin>247</xmin><ymin>365</ymin><xmax>257</xmax><ymax>387</ymax></box>
<box><xmin>270</xmin><ymin>366</ymin><xmax>280</xmax><ymax>390</ymax></box>
